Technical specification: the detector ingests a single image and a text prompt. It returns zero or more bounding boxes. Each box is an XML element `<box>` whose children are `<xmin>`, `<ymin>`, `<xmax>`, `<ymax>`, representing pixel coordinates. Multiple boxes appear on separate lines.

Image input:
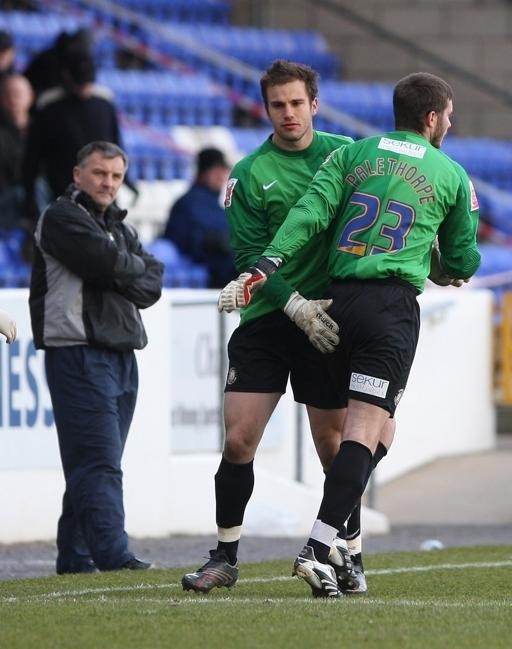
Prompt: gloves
<box><xmin>215</xmin><ymin>256</ymin><xmax>281</xmax><ymax>316</ymax></box>
<box><xmin>427</xmin><ymin>243</ymin><xmax>472</xmax><ymax>289</ymax></box>
<box><xmin>283</xmin><ymin>290</ymin><xmax>344</xmax><ymax>355</ymax></box>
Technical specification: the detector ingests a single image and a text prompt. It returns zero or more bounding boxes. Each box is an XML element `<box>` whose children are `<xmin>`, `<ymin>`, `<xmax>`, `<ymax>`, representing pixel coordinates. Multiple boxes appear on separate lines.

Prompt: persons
<box><xmin>219</xmin><ymin>69</ymin><xmax>485</xmax><ymax>597</ymax></box>
<box><xmin>28</xmin><ymin>138</ymin><xmax>163</xmax><ymax>575</ymax></box>
<box><xmin>163</xmin><ymin>146</ymin><xmax>235</xmax><ymax>289</ymax></box>
<box><xmin>0</xmin><ymin>311</ymin><xmax>18</xmax><ymax>345</ymax></box>
<box><xmin>180</xmin><ymin>56</ymin><xmax>469</xmax><ymax>597</ymax></box>
<box><xmin>0</xmin><ymin>24</ymin><xmax>141</xmax><ymax>260</ymax></box>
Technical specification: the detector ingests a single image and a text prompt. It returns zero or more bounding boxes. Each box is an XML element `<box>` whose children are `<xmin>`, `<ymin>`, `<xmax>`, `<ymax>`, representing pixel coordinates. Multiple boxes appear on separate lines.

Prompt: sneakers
<box><xmin>57</xmin><ymin>556</ymin><xmax>155</xmax><ymax>580</ymax></box>
<box><xmin>292</xmin><ymin>546</ymin><xmax>368</xmax><ymax>602</ymax></box>
<box><xmin>181</xmin><ymin>551</ymin><xmax>244</xmax><ymax>598</ymax></box>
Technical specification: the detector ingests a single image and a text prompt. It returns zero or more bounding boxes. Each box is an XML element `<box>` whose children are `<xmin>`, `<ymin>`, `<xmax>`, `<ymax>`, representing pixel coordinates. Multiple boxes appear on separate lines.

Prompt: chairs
<box><xmin>1</xmin><ymin>1</ymin><xmax>512</xmax><ymax>288</ymax></box>
